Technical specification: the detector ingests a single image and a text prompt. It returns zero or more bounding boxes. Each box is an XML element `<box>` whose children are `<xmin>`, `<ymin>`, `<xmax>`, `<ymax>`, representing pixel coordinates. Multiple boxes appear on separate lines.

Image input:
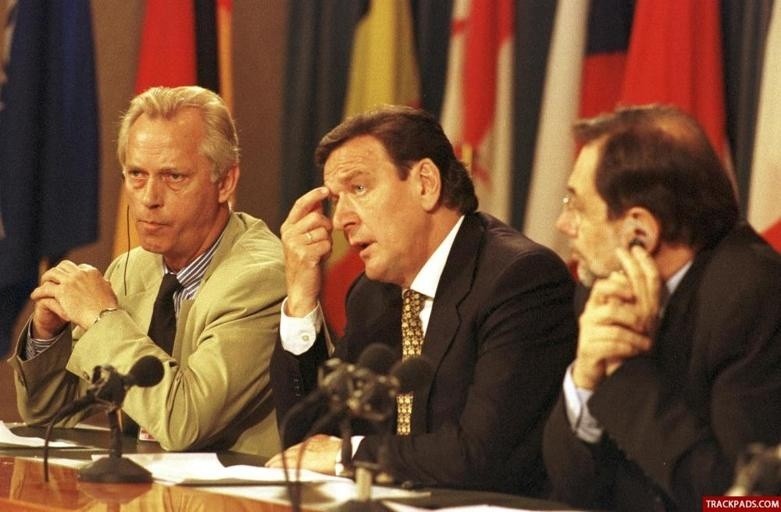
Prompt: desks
<box><xmin>2</xmin><ymin>424</ymin><xmax>590</xmax><ymax>510</ymax></box>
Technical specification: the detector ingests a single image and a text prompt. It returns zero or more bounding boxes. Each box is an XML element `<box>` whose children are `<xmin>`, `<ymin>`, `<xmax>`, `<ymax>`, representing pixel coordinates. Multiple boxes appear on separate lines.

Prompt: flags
<box><xmin>0</xmin><ymin>2</ymin><xmax>779</xmax><ymax>334</ymax></box>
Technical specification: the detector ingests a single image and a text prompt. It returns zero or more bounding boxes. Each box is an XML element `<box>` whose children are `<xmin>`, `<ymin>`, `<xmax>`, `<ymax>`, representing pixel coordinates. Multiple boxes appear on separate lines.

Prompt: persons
<box><xmin>5</xmin><ymin>85</ymin><xmax>336</xmax><ymax>460</ymax></box>
<box><xmin>539</xmin><ymin>102</ymin><xmax>780</xmax><ymax>511</ymax></box>
<box><xmin>269</xmin><ymin>104</ymin><xmax>578</xmax><ymax>502</ymax></box>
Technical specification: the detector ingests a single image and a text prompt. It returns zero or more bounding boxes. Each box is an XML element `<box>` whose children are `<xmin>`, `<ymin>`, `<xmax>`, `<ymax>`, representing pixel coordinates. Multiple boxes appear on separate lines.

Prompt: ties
<box><xmin>122</xmin><ymin>273</ymin><xmax>181</xmax><ymax>436</ymax></box>
<box><xmin>396</xmin><ymin>289</ymin><xmax>427</xmax><ymax>437</ymax></box>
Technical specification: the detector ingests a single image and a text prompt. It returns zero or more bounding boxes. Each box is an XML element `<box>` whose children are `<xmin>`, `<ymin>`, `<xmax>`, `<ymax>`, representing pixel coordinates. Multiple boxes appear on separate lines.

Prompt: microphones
<box><xmin>279</xmin><ymin>342</ymin><xmax>397</xmax><ymax>415</ymax></box>
<box><xmin>56</xmin><ymin>356</ymin><xmax>164</xmax><ymax>420</ymax></box>
<box><xmin>315</xmin><ymin>358</ymin><xmax>433</xmax><ymax>435</ymax></box>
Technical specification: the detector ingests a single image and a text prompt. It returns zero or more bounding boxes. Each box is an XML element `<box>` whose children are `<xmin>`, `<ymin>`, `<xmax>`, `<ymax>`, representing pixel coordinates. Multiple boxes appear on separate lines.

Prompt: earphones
<box><xmin>119</xmin><ymin>171</ymin><xmax>126</xmax><ymax>184</ymax></box>
<box><xmin>627</xmin><ymin>240</ymin><xmax>641</xmax><ymax>250</ymax></box>
